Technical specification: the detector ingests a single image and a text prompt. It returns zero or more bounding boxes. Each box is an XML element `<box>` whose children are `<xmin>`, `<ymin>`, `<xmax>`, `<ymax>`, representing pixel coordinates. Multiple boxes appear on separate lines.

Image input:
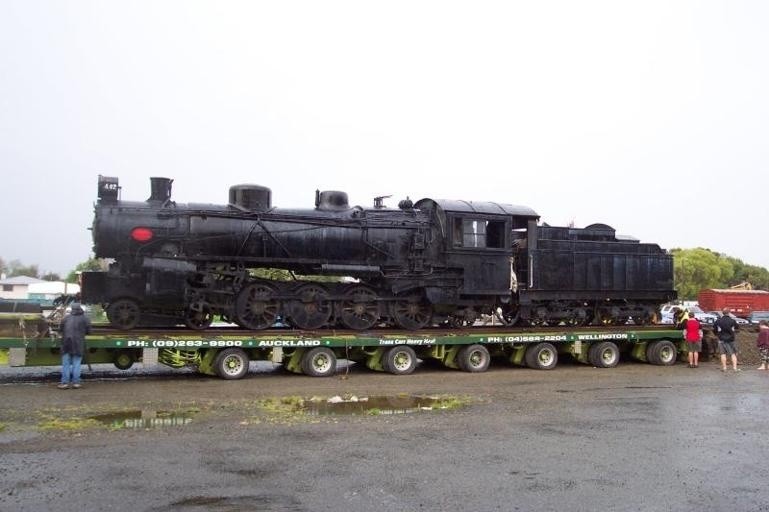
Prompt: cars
<box><xmin>662</xmin><ymin>304</ymin><xmax>769</xmax><ymax>327</ymax></box>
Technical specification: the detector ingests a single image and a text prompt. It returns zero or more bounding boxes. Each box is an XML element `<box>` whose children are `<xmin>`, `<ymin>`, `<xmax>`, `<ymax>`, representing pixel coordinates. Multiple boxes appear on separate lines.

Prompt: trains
<box><xmin>81</xmin><ymin>176</ymin><xmax>679</xmax><ymax>330</ymax></box>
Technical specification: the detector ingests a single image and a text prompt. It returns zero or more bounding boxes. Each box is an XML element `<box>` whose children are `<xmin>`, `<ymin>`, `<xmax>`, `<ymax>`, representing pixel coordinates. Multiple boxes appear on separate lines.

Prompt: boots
<box><xmin>758</xmin><ymin>363</ymin><xmax>765</xmax><ymax>370</ymax></box>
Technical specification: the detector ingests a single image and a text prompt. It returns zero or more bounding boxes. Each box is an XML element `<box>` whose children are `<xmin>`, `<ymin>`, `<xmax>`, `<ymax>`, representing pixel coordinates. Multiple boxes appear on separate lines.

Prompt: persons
<box><xmin>712</xmin><ymin>307</ymin><xmax>741</xmax><ymax>373</ymax></box>
<box><xmin>757</xmin><ymin>320</ymin><xmax>769</xmax><ymax>370</ymax></box>
<box><xmin>683</xmin><ymin>312</ymin><xmax>703</xmax><ymax>368</ymax></box>
<box><xmin>57</xmin><ymin>303</ymin><xmax>90</xmax><ymax>389</ymax></box>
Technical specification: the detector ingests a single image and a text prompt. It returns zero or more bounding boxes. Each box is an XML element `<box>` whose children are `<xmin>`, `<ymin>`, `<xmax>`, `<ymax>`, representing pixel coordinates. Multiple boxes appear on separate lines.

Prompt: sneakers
<box><xmin>687</xmin><ymin>364</ymin><xmax>693</xmax><ymax>368</ymax></box>
<box><xmin>694</xmin><ymin>365</ymin><xmax>699</xmax><ymax>368</ymax></box>
<box><xmin>721</xmin><ymin>369</ymin><xmax>726</xmax><ymax>372</ymax></box>
<box><xmin>73</xmin><ymin>383</ymin><xmax>82</xmax><ymax>389</ymax></box>
<box><xmin>57</xmin><ymin>384</ymin><xmax>68</xmax><ymax>389</ymax></box>
<box><xmin>734</xmin><ymin>369</ymin><xmax>741</xmax><ymax>372</ymax></box>
